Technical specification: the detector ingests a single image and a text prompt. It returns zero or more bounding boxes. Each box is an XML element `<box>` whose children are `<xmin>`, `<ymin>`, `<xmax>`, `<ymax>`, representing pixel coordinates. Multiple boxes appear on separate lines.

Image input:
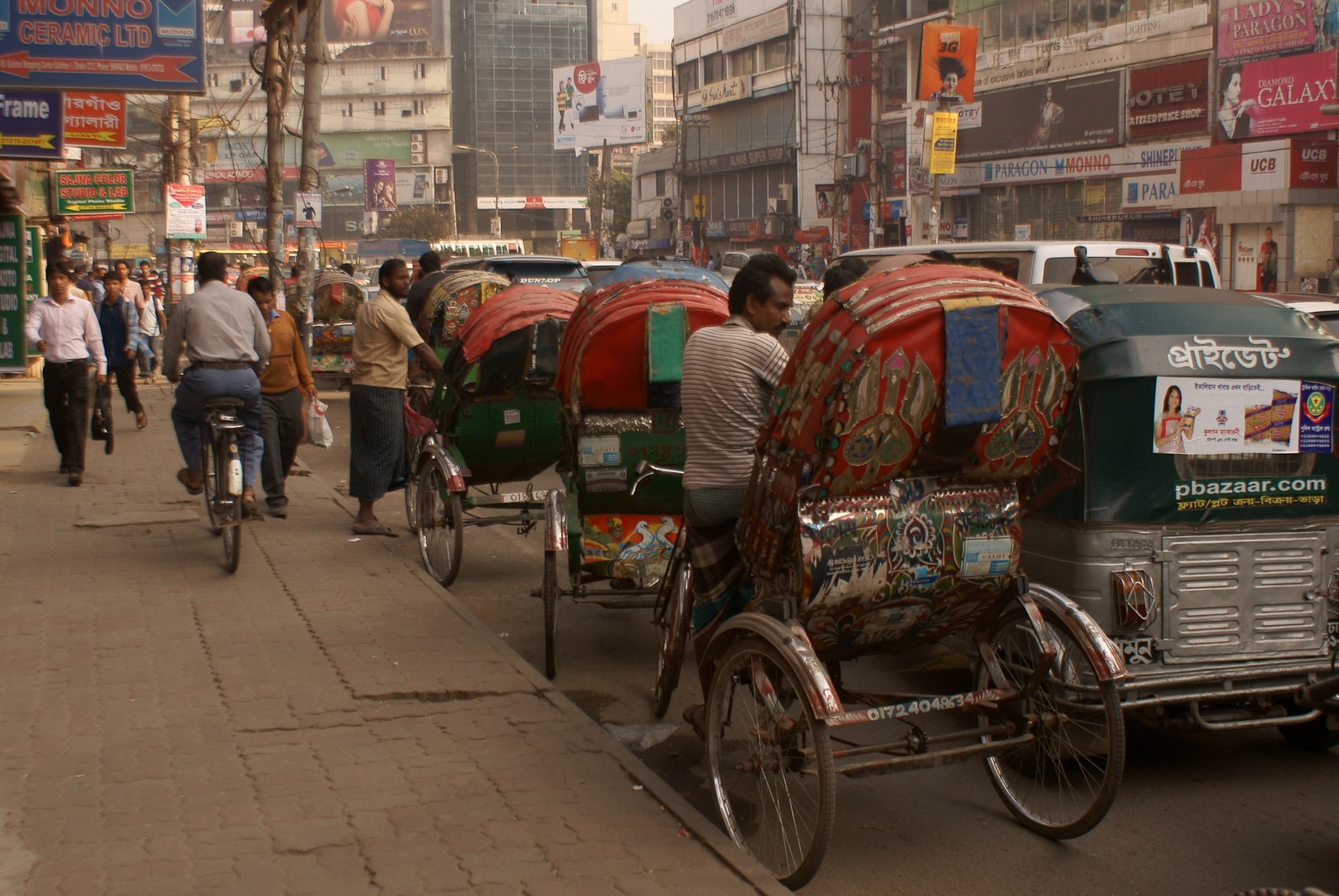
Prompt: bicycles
<box><xmin>170</xmin><ymin>374</ymin><xmax>246</xmax><ymax>574</ymax></box>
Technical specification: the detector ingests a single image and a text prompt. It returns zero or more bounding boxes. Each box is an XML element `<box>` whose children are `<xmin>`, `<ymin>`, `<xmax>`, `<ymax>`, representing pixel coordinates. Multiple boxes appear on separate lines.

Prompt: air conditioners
<box><xmin>435</xmin><ymin>185</ymin><xmax>447</xmax><ymax>201</ymax></box>
<box><xmin>768</xmin><ymin>198</ymin><xmax>789</xmax><ymax>213</ymax></box>
<box><xmin>663</xmin><ymin>209</ymin><xmax>678</xmax><ymax>220</ymax></box>
<box><xmin>662</xmin><ymin>195</ymin><xmax>679</xmax><ymax>208</ymax></box>
<box><xmin>412</xmin><ymin>134</ymin><xmax>423</xmax><ymax>142</ymax></box>
<box><xmin>411</xmin><ymin>153</ymin><xmax>422</xmax><ymax>164</ymax></box>
<box><xmin>435</xmin><ymin>168</ymin><xmax>448</xmax><ymax>183</ymax></box>
<box><xmin>251</xmin><ymin>228</ymin><xmax>264</xmax><ymax>243</ymax></box>
<box><xmin>411</xmin><ymin>142</ymin><xmax>423</xmax><ymax>152</ymax></box>
<box><xmin>765</xmin><ymin>220</ymin><xmax>784</xmax><ymax>235</ymax></box>
<box><xmin>230</xmin><ymin>221</ymin><xmax>243</xmax><ymax>237</ymax></box>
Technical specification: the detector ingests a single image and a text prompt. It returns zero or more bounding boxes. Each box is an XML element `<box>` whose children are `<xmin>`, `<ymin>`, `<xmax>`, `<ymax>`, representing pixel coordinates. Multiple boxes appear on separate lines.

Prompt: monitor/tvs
<box><xmin>578</xmin><ymin>105</ymin><xmax>600</xmax><ymax>123</ymax></box>
<box><xmin>627</xmin><ymin>111</ymin><xmax>640</xmax><ymax>120</ymax></box>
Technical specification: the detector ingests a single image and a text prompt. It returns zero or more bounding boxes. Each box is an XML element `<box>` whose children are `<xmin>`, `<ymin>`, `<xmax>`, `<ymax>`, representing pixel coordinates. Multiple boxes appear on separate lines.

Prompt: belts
<box><xmin>193</xmin><ymin>361</ymin><xmax>250</xmax><ymax>371</ymax></box>
<box><xmin>47</xmin><ymin>359</ymin><xmax>85</xmax><ymax>370</ymax></box>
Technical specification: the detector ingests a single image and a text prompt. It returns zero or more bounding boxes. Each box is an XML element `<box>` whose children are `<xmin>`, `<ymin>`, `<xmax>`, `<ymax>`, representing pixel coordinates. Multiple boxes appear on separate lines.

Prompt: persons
<box><xmin>818</xmin><ymin>192</ymin><xmax>836</xmax><ymax>218</ymax></box>
<box><xmin>682</xmin><ymin>255</ymin><xmax>793</xmax><ymax>742</ymax></box>
<box><xmin>1217</xmin><ymin>63</ymin><xmax>1255</xmax><ymax>139</ymax></box>
<box><xmin>927</xmin><ymin>57</ymin><xmax>966</xmax><ymax>103</ymax></box>
<box><xmin>302</xmin><ymin>203</ymin><xmax>317</xmax><ymax>221</ymax></box>
<box><xmin>1260</xmin><ymin>226</ymin><xmax>1278</xmax><ymax>251</ymax></box>
<box><xmin>367</xmin><ymin>180</ymin><xmax>396</xmax><ymax>208</ymax></box>
<box><xmin>1154</xmin><ymin>385</ymin><xmax>1201</xmax><ymax>452</ymax></box>
<box><xmin>325</xmin><ymin>0</ymin><xmax>395</xmax><ymax>42</ymax></box>
<box><xmin>556</xmin><ymin>77</ymin><xmax>575</xmax><ymax>134</ymax></box>
<box><xmin>1327</xmin><ymin>258</ymin><xmax>1339</xmax><ymax>296</ymax></box>
<box><xmin>194</xmin><ymin>219</ymin><xmax>203</xmax><ymax>234</ymax></box>
<box><xmin>1194</xmin><ymin>212</ymin><xmax>1218</xmax><ymax>261</ymax></box>
<box><xmin>1260</xmin><ymin>242</ymin><xmax>1277</xmax><ymax>291</ymax></box>
<box><xmin>23</xmin><ymin>243</ymin><xmax>870</xmax><ymax>538</ymax></box>
<box><xmin>1034</xmin><ymin>85</ymin><xmax>1065</xmax><ymax>147</ymax></box>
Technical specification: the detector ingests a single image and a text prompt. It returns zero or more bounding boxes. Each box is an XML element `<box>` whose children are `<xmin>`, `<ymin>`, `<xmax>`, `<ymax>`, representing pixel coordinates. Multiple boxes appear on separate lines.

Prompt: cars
<box><xmin>1240</xmin><ymin>291</ymin><xmax>1339</xmax><ymax>337</ymax></box>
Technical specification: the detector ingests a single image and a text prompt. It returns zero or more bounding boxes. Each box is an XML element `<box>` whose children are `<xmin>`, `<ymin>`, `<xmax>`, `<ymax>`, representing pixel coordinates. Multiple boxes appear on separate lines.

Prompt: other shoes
<box><xmin>270</xmin><ymin>504</ymin><xmax>288</xmax><ymax>517</ymax></box>
<box><xmin>68</xmin><ymin>473</ymin><xmax>82</xmax><ymax>486</ymax></box>
<box><xmin>59</xmin><ymin>463</ymin><xmax>68</xmax><ymax>474</ymax></box>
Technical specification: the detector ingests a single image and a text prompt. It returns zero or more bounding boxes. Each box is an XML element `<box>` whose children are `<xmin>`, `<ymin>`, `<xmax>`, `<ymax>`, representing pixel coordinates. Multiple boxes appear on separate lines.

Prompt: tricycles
<box><xmin>405</xmin><ymin>259</ymin><xmax>1130</xmax><ymax>893</ymax></box>
<box><xmin>237</xmin><ymin>267</ymin><xmax>365</xmax><ymax>390</ymax></box>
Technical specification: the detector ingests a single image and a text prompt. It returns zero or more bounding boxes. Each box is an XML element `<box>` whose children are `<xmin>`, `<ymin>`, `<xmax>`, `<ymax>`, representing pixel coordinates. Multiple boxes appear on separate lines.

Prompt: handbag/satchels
<box><xmin>303</xmin><ymin>396</ymin><xmax>334</xmax><ymax>449</ymax></box>
<box><xmin>92</xmin><ymin>384</ymin><xmax>108</xmax><ymax>440</ymax></box>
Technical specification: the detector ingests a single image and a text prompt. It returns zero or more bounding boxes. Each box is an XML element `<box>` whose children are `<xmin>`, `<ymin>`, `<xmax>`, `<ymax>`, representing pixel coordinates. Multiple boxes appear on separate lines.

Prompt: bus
<box><xmin>192</xmin><ymin>248</ymin><xmax>301</xmax><ymax>288</ymax></box>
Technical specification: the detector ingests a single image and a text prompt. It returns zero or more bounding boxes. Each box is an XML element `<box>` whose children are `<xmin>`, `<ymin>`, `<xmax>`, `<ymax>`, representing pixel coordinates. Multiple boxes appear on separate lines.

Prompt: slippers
<box><xmin>177</xmin><ymin>468</ymin><xmax>203</xmax><ymax>494</ymax></box>
<box><xmin>682</xmin><ymin>703</ymin><xmax>707</xmax><ymax>741</ymax></box>
<box><xmin>352</xmin><ymin>522</ymin><xmax>399</xmax><ymax>537</ymax></box>
<box><xmin>241</xmin><ymin>500</ymin><xmax>256</xmax><ymax>517</ymax></box>
<box><xmin>137</xmin><ymin>405</ymin><xmax>148</xmax><ymax>429</ymax></box>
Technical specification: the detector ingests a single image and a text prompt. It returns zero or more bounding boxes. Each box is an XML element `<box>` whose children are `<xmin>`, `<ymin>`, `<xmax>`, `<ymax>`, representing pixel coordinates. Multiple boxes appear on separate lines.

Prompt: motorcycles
<box><xmin>962</xmin><ymin>280</ymin><xmax>1339</xmax><ymax>764</ymax></box>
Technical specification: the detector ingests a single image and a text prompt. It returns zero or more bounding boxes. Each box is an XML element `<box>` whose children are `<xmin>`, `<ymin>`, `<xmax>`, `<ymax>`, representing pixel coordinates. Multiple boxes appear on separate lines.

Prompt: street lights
<box><xmin>455</xmin><ymin>144</ymin><xmax>500</xmax><ymax>237</ymax></box>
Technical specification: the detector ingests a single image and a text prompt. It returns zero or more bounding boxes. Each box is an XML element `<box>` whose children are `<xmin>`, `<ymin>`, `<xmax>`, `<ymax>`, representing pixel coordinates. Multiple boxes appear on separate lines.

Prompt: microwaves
<box><xmin>627</xmin><ymin>110</ymin><xmax>641</xmax><ymax>120</ymax></box>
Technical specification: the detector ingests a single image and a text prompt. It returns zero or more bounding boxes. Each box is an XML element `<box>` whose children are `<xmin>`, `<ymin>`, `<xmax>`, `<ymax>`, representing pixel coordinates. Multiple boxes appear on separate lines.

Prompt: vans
<box><xmin>357</xmin><ymin>263</ymin><xmax>416</xmax><ymax>305</ymax></box>
<box><xmin>721</xmin><ymin>251</ymin><xmax>769</xmax><ymax>276</ymax></box>
<box><xmin>576</xmin><ymin>259</ymin><xmax>625</xmax><ymax>286</ymax></box>
<box><xmin>817</xmin><ymin>239</ymin><xmax>1223</xmax><ymax>290</ymax></box>
<box><xmin>439</xmin><ymin>255</ymin><xmax>592</xmax><ymax>300</ymax></box>
